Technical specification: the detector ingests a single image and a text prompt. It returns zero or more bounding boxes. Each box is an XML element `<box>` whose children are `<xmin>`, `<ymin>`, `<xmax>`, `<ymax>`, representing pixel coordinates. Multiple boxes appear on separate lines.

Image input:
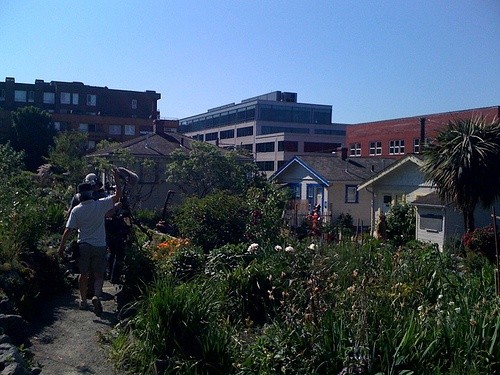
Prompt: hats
<box><xmin>77</xmin><ymin>183</ymin><xmax>93</xmax><ymax>193</ymax></box>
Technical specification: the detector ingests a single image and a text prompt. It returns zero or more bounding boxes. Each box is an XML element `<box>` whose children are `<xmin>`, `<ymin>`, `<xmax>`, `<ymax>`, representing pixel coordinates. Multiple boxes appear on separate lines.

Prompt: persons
<box><xmin>68</xmin><ymin>173</ymin><xmax>128</xmax><ymax>283</ymax></box>
<box><xmin>58</xmin><ymin>171</ymin><xmax>122</xmax><ymax>315</ymax></box>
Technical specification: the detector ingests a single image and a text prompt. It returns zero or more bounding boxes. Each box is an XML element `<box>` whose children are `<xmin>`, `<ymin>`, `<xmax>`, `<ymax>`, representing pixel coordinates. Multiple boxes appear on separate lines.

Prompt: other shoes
<box><xmin>92</xmin><ymin>297</ymin><xmax>103</xmax><ymax>315</ymax></box>
<box><xmin>79</xmin><ymin>300</ymin><xmax>87</xmax><ymax>308</ymax></box>
<box><xmin>110</xmin><ymin>279</ymin><xmax>117</xmax><ymax>283</ymax></box>
<box><xmin>106</xmin><ymin>272</ymin><xmax>110</xmax><ymax>277</ymax></box>
